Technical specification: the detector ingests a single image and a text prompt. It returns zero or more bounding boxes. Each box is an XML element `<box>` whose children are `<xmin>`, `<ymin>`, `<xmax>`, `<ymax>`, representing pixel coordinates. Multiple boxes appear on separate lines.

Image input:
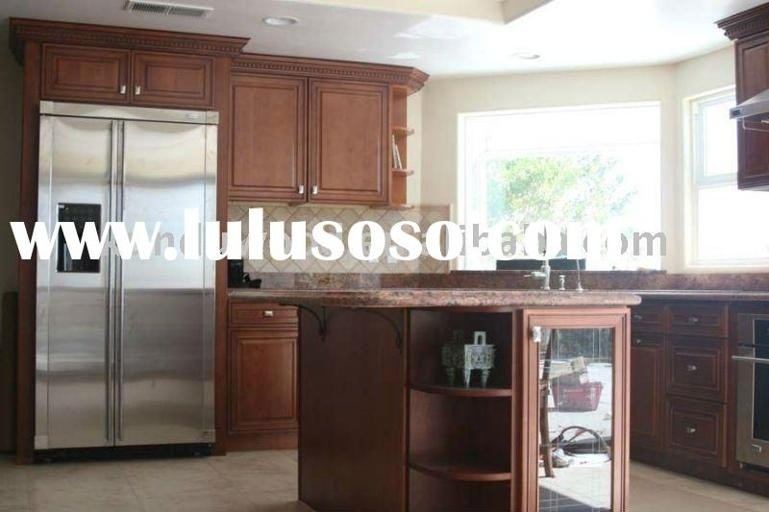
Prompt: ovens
<box><xmin>731</xmin><ymin>311</ymin><xmax>769</xmax><ymax>473</ymax></box>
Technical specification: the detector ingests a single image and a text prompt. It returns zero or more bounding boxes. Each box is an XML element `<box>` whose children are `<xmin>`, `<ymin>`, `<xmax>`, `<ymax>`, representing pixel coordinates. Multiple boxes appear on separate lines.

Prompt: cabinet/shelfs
<box><xmin>8</xmin><ymin>2</ymin><xmax>769</xmax><ymax>512</ymax></box>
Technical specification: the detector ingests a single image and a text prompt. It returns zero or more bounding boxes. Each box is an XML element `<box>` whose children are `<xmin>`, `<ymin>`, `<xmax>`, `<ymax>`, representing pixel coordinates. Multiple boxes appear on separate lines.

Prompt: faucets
<box><xmin>530</xmin><ymin>259</ymin><xmax>552</xmax><ymax>290</ymax></box>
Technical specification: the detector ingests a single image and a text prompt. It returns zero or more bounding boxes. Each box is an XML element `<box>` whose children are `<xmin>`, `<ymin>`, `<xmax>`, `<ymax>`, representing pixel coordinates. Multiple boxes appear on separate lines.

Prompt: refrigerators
<box><xmin>30</xmin><ymin>99</ymin><xmax>220</xmax><ymax>452</ymax></box>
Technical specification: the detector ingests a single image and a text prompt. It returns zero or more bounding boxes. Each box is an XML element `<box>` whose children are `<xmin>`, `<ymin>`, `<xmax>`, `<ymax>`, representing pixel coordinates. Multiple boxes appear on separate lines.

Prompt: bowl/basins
<box><xmin>551</xmin><ymin>383</ymin><xmax>602</xmax><ymax>412</ymax></box>
